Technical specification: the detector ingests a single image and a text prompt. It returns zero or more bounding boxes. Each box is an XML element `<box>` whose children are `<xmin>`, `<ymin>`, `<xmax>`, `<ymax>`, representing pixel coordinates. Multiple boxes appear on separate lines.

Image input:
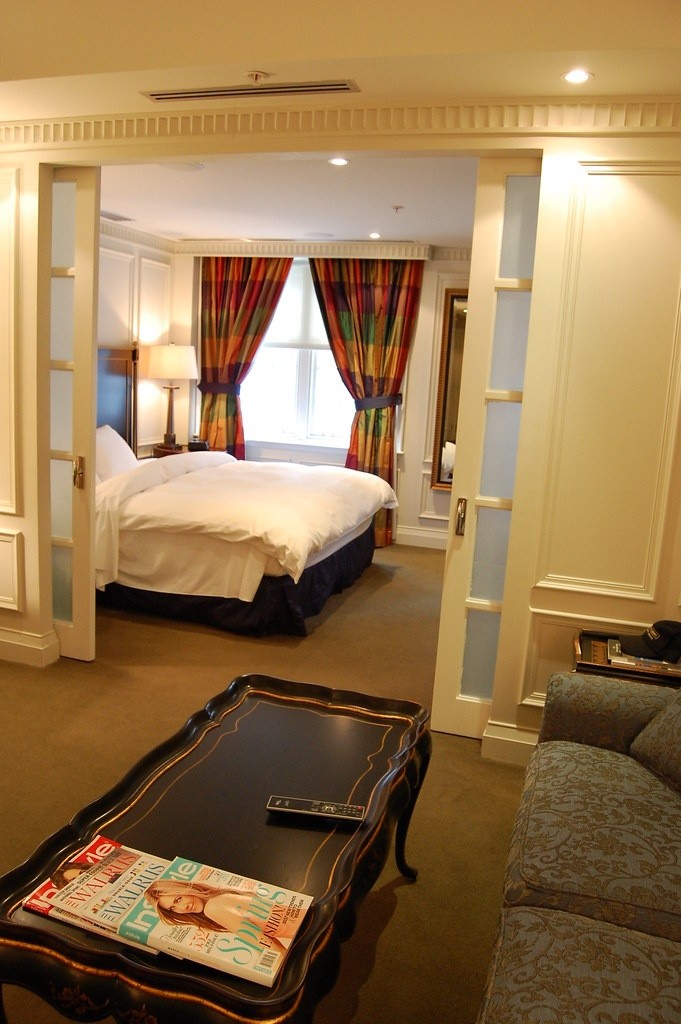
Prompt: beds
<box><xmin>94</xmin><ymin>458</ymin><xmax>375</xmax><ymax>636</ymax></box>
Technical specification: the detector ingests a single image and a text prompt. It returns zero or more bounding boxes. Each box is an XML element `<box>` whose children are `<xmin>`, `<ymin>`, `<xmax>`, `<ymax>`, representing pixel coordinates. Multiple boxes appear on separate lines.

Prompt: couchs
<box><xmin>476</xmin><ymin>671</ymin><xmax>680</xmax><ymax>1024</ymax></box>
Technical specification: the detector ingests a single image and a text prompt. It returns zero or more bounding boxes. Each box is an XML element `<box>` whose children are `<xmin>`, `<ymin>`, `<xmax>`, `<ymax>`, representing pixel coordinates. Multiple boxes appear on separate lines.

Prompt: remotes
<box><xmin>267</xmin><ymin>796</ymin><xmax>365</xmax><ymax>821</ymax></box>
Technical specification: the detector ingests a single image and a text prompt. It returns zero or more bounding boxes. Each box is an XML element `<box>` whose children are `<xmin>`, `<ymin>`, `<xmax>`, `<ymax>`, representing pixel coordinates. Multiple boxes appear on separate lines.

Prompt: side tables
<box><xmin>573</xmin><ymin>628</ymin><xmax>680</xmax><ymax>688</ymax></box>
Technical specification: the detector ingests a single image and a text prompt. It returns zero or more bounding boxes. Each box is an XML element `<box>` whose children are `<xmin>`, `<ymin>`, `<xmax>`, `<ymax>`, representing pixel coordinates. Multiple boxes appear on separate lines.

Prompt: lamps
<box><xmin>149</xmin><ymin>342</ymin><xmax>199</xmax><ymax>458</ymax></box>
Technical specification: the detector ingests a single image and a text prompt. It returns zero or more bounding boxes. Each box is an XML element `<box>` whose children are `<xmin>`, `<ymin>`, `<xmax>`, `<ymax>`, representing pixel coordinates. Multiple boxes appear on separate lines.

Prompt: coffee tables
<box><xmin>0</xmin><ymin>672</ymin><xmax>433</xmax><ymax>1023</ymax></box>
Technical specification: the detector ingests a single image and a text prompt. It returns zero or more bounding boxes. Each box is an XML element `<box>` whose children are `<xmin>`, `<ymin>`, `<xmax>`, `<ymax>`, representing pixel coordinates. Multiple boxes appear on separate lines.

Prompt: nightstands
<box><xmin>139</xmin><ymin>446</ymin><xmax>228</xmax><ymax>460</ymax></box>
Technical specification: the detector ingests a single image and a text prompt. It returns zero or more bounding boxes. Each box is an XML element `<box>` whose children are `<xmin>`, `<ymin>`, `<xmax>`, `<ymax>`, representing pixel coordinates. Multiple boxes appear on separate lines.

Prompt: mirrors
<box><xmin>430</xmin><ymin>287</ymin><xmax>469</xmax><ymax>491</ymax></box>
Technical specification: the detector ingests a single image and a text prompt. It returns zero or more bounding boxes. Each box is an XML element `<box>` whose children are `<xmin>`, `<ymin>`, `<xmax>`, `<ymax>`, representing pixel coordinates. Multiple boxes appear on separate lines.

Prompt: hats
<box><xmin>620</xmin><ymin>619</ymin><xmax>681</xmax><ymax>666</ymax></box>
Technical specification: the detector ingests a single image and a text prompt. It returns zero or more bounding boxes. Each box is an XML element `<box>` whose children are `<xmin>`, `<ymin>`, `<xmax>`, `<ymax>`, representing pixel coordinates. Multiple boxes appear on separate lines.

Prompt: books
<box><xmin>606</xmin><ymin>640</ymin><xmax>681</xmax><ymax>676</ymax></box>
<box><xmin>21</xmin><ymin>833</ymin><xmax>314</xmax><ymax>988</ymax></box>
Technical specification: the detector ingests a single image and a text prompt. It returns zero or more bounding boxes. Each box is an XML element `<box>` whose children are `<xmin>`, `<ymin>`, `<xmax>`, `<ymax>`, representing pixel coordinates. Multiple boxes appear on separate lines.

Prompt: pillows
<box><xmin>95</xmin><ymin>424</ymin><xmax>138</xmax><ymax>482</ymax></box>
